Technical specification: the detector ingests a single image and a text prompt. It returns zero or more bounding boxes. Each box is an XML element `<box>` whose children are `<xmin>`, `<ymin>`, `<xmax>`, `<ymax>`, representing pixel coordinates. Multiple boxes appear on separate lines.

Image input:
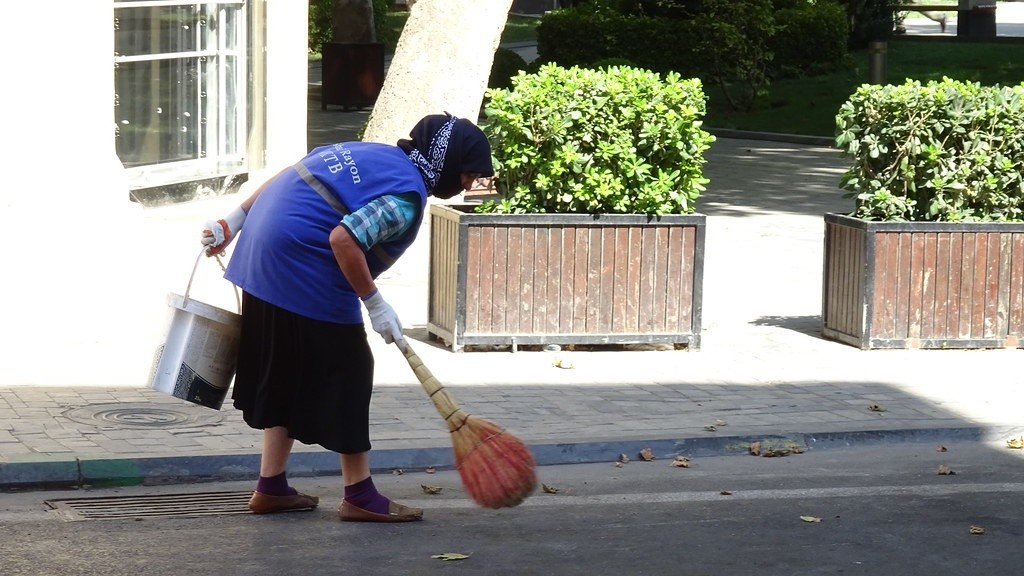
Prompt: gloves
<box><xmin>360</xmin><ymin>288</ymin><xmax>409</xmax><ymax>354</ymax></box>
<box><xmin>201</xmin><ymin>206</ymin><xmax>247</xmax><ymax>257</ymax></box>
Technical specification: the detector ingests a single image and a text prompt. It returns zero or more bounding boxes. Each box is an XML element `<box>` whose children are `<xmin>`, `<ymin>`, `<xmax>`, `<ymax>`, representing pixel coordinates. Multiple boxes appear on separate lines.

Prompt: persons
<box><xmin>200</xmin><ymin>112</ymin><xmax>495</xmax><ymax>521</ymax></box>
<box><xmin>892</xmin><ymin>0</ymin><xmax>946</xmax><ymax>33</ymax></box>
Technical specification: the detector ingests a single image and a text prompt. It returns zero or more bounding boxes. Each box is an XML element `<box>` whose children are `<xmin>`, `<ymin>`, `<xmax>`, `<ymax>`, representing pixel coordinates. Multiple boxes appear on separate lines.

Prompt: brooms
<box><xmin>390</xmin><ymin>334</ymin><xmax>539</xmax><ymax>511</ymax></box>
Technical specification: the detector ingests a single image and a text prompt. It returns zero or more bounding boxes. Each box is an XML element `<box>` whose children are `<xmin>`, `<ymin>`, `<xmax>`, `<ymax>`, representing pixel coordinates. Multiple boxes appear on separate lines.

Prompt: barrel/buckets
<box><xmin>147</xmin><ymin>245</ymin><xmax>245</xmax><ymax>412</ymax></box>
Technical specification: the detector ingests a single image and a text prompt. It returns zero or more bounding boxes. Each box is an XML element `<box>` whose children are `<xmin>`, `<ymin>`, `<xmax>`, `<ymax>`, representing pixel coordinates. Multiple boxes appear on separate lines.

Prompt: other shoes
<box><xmin>338</xmin><ymin>497</ymin><xmax>424</xmax><ymax>522</ymax></box>
<box><xmin>248</xmin><ymin>487</ymin><xmax>319</xmax><ymax>511</ymax></box>
